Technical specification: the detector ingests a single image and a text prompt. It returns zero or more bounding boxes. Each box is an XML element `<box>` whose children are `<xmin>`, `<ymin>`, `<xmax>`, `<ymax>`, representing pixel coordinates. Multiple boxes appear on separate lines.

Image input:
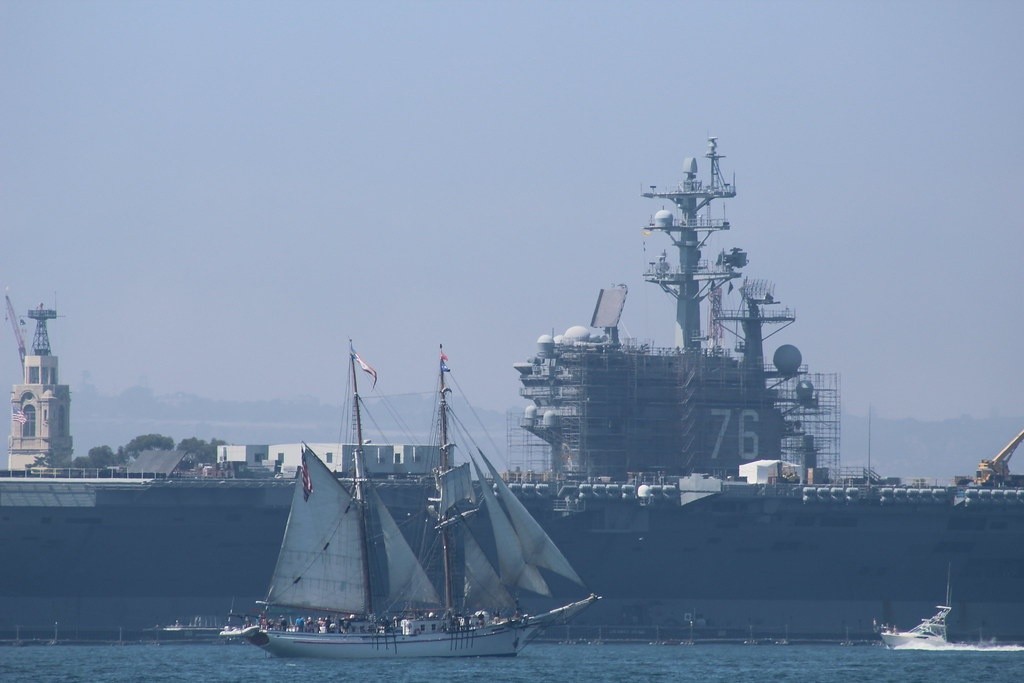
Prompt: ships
<box><xmin>0</xmin><ymin>131</ymin><xmax>1024</xmax><ymax>648</ymax></box>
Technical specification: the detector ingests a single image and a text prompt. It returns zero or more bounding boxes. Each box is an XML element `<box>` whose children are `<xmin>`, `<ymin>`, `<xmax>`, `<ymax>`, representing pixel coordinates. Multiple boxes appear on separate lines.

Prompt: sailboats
<box><xmin>217</xmin><ymin>337</ymin><xmax>604</xmax><ymax>660</ymax></box>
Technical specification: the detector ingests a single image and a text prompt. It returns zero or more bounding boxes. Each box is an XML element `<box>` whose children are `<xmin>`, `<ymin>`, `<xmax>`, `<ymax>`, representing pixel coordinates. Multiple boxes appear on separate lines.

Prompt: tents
<box><xmin>739</xmin><ymin>459</ymin><xmax>803</xmax><ymax>484</ymax></box>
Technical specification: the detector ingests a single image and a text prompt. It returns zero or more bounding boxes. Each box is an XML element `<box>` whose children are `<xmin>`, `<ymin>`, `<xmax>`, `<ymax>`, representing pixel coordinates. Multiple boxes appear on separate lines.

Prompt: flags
<box><xmin>350</xmin><ymin>349</ymin><xmax>377</xmax><ymax>391</ymax></box>
<box><xmin>440</xmin><ymin>359</ymin><xmax>450</xmax><ymax>372</ymax></box>
<box><xmin>301</xmin><ymin>447</ymin><xmax>314</xmax><ymax>503</ymax></box>
<box><xmin>12</xmin><ymin>407</ymin><xmax>28</xmax><ymax>424</ymax></box>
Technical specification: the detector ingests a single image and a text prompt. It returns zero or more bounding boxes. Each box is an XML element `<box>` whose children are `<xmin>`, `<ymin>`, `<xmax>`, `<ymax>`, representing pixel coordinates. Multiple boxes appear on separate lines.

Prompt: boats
<box><xmin>141</xmin><ymin>616</ymin><xmax>243</xmax><ymax>642</ymax></box>
<box><xmin>872</xmin><ymin>564</ymin><xmax>998</xmax><ymax>650</ymax></box>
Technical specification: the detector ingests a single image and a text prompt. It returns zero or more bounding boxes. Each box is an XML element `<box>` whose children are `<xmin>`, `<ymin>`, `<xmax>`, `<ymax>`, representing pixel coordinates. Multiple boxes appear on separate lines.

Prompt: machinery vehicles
<box><xmin>972</xmin><ymin>429</ymin><xmax>1024</xmax><ymax>486</ymax></box>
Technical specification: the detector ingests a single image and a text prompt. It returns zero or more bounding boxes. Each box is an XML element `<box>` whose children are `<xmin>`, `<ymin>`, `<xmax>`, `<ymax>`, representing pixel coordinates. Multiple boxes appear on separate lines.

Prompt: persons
<box><xmin>268</xmin><ymin>615</ymin><xmax>350</xmax><ymax>634</ymax></box>
